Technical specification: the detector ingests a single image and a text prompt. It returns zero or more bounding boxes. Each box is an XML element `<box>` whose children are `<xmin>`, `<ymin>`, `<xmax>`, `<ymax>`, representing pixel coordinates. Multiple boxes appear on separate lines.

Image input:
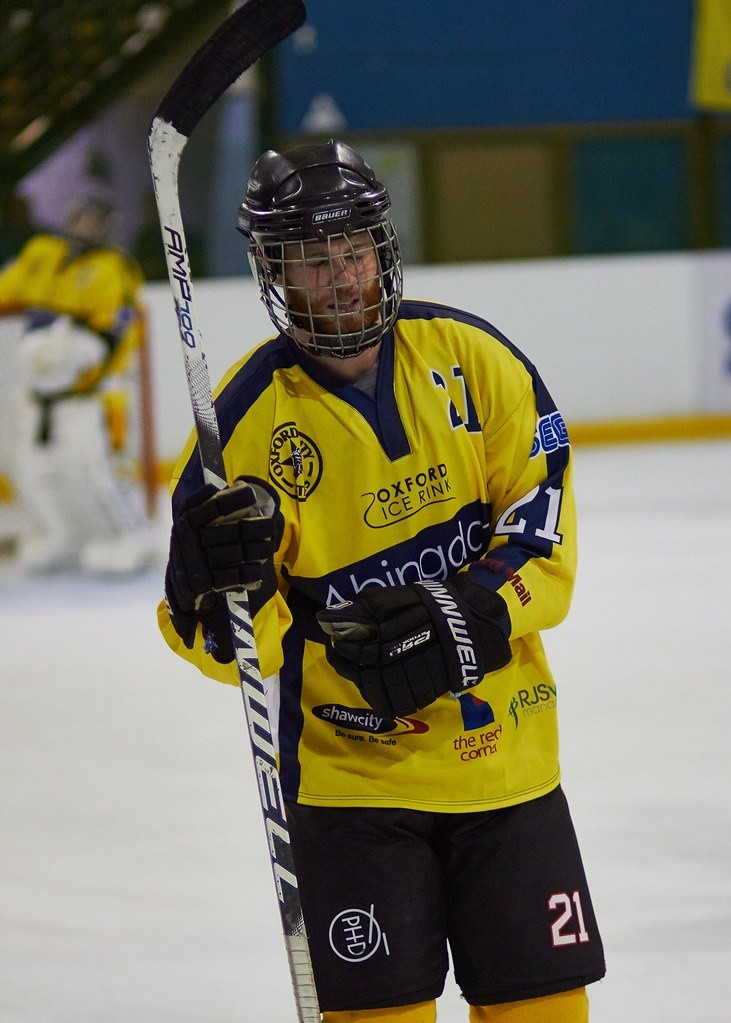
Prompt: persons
<box><xmin>156</xmin><ymin>141</ymin><xmax>608</xmax><ymax>1022</ymax></box>
<box><xmin>0</xmin><ymin>195</ymin><xmax>165</xmax><ymax>579</ymax></box>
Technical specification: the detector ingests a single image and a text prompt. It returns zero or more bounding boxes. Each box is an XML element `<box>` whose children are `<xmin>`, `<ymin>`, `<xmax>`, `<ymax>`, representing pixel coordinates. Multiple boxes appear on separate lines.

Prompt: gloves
<box><xmin>316</xmin><ymin>572</ymin><xmax>513</xmax><ymax>717</ymax></box>
<box><xmin>163</xmin><ymin>474</ymin><xmax>285</xmax><ymax>664</ymax></box>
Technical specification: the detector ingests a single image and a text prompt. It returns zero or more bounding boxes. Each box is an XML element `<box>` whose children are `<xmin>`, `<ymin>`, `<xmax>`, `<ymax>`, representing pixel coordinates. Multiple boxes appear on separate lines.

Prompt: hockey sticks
<box><xmin>142</xmin><ymin>0</ymin><xmax>327</xmax><ymax>1023</ymax></box>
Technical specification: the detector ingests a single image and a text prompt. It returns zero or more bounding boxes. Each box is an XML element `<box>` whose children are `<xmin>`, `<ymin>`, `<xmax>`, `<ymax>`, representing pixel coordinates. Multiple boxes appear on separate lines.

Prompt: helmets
<box><xmin>235</xmin><ymin>139</ymin><xmax>404</xmax><ymax>359</ymax></box>
